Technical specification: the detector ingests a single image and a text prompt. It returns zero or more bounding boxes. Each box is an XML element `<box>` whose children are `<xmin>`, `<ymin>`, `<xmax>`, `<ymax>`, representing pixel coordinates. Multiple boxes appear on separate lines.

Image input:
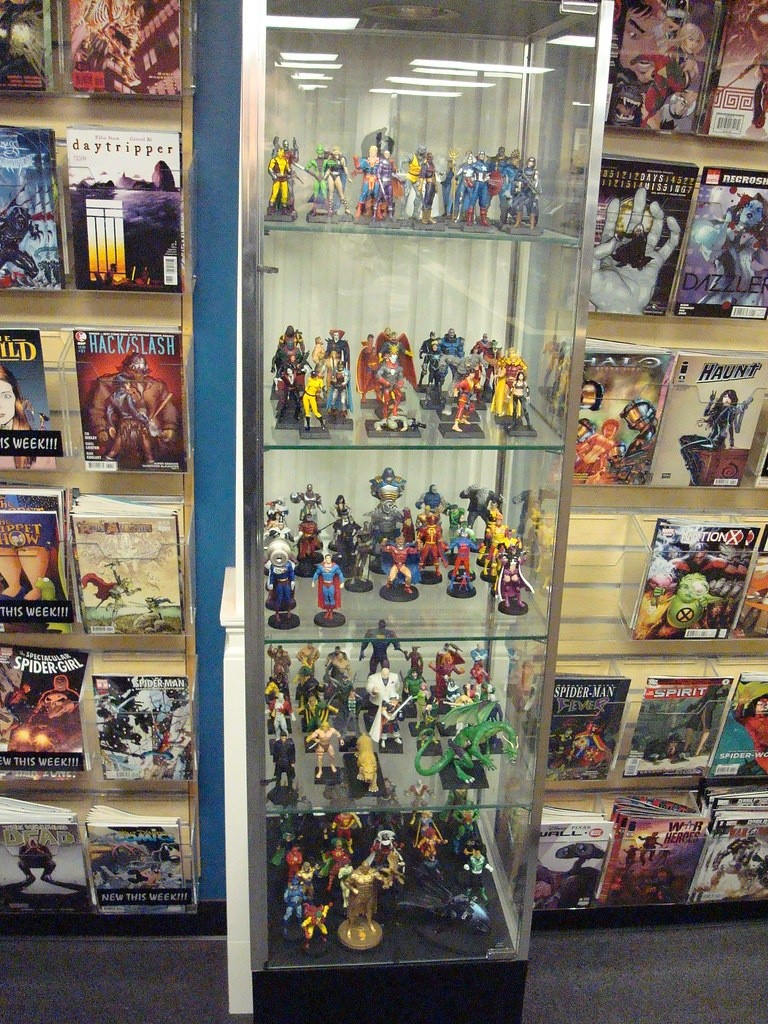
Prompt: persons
<box><xmin>266</xmin><ymin>550</ymin><xmax>297</xmax><ymax>624</ymax></box>
<box><xmin>265</xmin><ymin>620</ymin><xmax>504</xmax><ymax>947</ymax></box>
<box><xmin>270</xmin><ymin>326</ymin><xmax>536</xmax><ymax>431</ymax></box>
<box><xmin>267</xmin><ymin>134</ymin><xmax>542</xmax><ymax>232</ymax></box>
<box><xmin>263</xmin><ymin>467</ymin><xmax>557</xmax><ymax>606</ymax></box>
<box><xmin>310</xmin><ymin>555</ymin><xmax>345</xmax><ymax>620</ymax></box>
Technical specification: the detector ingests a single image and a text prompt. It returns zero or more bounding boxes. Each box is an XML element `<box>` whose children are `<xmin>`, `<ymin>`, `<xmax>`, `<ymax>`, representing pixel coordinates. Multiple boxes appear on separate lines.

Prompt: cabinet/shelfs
<box><xmin>0</xmin><ymin>0</ymin><xmax>199</xmax><ymax>933</ymax></box>
<box><xmin>243</xmin><ymin>21</ymin><xmax>613</xmax><ymax>968</ymax></box>
<box><xmin>530</xmin><ymin>0</ymin><xmax>768</xmax><ymax>914</ymax></box>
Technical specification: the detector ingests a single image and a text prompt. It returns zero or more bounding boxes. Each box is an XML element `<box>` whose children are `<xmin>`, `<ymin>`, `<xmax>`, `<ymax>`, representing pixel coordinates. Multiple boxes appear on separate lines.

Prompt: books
<box><xmin>0</xmin><ymin>643</ymin><xmax>194</xmax><ymax>783</ymax></box>
<box><xmin>0</xmin><ymin>796</ymin><xmax>186</xmax><ymax>920</ymax></box>
<box><xmin>0</xmin><ymin>0</ymin><xmax>182</xmax><ymax>96</ymax></box>
<box><xmin>508</xmin><ymin>786</ymin><xmax>768</xmax><ymax>911</ymax></box>
<box><xmin>525</xmin><ymin>671</ymin><xmax>768</xmax><ymax>778</ymax></box>
<box><xmin>565</xmin><ymin>153</ymin><xmax>768</xmax><ymax>320</ymax></box>
<box><xmin>0</xmin><ymin>125</ymin><xmax>184</xmax><ymax>293</ymax></box>
<box><xmin>0</xmin><ymin>327</ymin><xmax>189</xmax><ymax>475</ymax></box>
<box><xmin>632</xmin><ymin>517</ymin><xmax>768</xmax><ymax>640</ymax></box>
<box><xmin>593</xmin><ymin>0</ymin><xmax>768</xmax><ymax>144</ymax></box>
<box><xmin>0</xmin><ymin>487</ymin><xmax>185</xmax><ymax>633</ymax></box>
<box><xmin>547</xmin><ymin>338</ymin><xmax>768</xmax><ymax>487</ymax></box>
